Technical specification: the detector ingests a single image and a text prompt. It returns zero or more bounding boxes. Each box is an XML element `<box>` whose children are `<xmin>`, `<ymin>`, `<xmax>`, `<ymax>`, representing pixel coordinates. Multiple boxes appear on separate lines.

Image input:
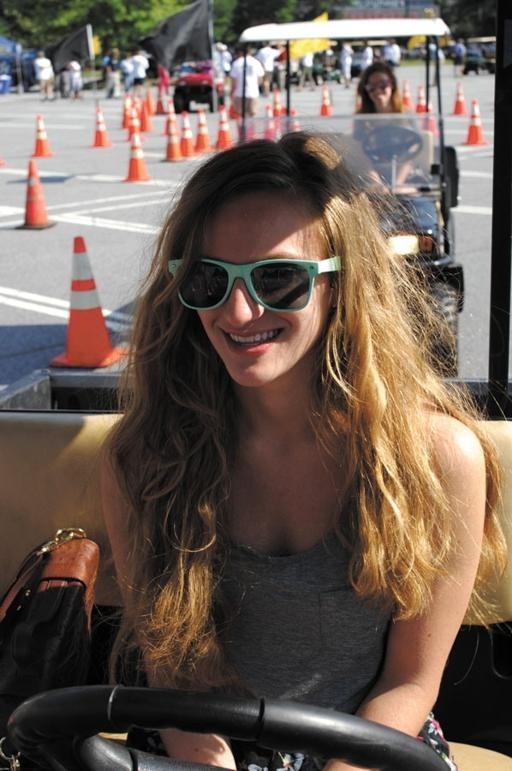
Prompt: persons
<box><xmin>35</xmin><ymin>39</ymin><xmax>497</xmax><ymax>197</ymax></box>
<box><xmin>99</xmin><ymin>130</ymin><xmax>509</xmax><ymax>771</ymax></box>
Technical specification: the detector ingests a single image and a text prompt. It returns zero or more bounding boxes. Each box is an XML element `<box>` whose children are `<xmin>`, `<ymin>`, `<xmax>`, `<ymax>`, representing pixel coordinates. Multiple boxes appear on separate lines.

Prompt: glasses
<box><xmin>363</xmin><ymin>79</ymin><xmax>392</xmax><ymax>94</ymax></box>
<box><xmin>168</xmin><ymin>254</ymin><xmax>342</xmax><ymax>313</ymax></box>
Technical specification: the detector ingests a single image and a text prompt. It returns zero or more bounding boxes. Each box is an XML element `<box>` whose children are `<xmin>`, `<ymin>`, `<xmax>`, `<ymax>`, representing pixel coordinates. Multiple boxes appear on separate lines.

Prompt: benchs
<box><xmin>300</xmin><ymin>131</ymin><xmax>433</xmax><ymax>182</ymax></box>
<box><xmin>0</xmin><ymin>411</ymin><xmax>512</xmax><ymax>756</ymax></box>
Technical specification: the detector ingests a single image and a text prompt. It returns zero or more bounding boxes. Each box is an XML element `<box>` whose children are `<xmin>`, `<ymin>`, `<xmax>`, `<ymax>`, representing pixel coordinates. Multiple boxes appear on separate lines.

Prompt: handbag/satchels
<box><xmin>0</xmin><ymin>526</ymin><xmax>104</xmax><ymax>704</ymax></box>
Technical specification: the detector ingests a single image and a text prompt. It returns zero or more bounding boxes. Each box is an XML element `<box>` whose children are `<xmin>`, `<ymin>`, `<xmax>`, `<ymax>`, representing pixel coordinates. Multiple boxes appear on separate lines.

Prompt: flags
<box><xmin>43</xmin><ymin>23</ymin><xmax>95</xmax><ymax>74</ymax></box>
<box><xmin>136</xmin><ymin>0</ymin><xmax>216</xmax><ymax>71</ymax></box>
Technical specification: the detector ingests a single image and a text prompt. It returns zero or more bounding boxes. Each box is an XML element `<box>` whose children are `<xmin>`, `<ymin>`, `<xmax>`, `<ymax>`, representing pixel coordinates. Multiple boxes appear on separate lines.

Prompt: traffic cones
<box><xmin>13</xmin><ymin>155</ymin><xmax>61</xmax><ymax>234</ymax></box>
<box><xmin>45</xmin><ymin>230</ymin><xmax>134</xmax><ymax>373</ymax></box>
